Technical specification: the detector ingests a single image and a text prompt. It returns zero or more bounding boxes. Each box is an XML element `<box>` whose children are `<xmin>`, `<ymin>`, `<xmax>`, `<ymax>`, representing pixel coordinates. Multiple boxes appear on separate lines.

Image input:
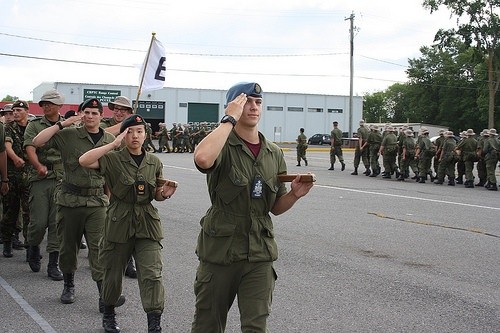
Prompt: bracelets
<box><xmin>1</xmin><ymin>180</ymin><xmax>9</xmax><ymax>183</ymax></box>
<box><xmin>161</xmin><ymin>191</ymin><xmax>170</xmax><ymax>199</ymax></box>
<box><xmin>56</xmin><ymin>121</ymin><xmax>63</xmax><ymax>130</ymax></box>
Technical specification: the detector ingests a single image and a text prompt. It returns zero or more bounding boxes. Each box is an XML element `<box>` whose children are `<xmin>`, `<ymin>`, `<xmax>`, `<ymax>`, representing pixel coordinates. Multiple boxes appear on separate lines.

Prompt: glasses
<box><xmin>41</xmin><ymin>101</ymin><xmax>53</xmax><ymax>107</ymax></box>
<box><xmin>114</xmin><ymin>107</ymin><xmax>129</xmax><ymax>113</ymax></box>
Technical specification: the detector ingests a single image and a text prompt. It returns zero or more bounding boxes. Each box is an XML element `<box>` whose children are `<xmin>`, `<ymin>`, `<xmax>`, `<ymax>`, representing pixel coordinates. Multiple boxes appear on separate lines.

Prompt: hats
<box><xmin>38</xmin><ymin>89</ymin><xmax>65</xmax><ymax>107</ymax></box>
<box><xmin>78</xmin><ymin>101</ymin><xmax>84</xmax><ymax>112</ymax></box>
<box><xmin>359</xmin><ymin>119</ymin><xmax>499</xmax><ymax>137</ymax></box>
<box><xmin>147</xmin><ymin>122</ymin><xmax>221</xmax><ymax>128</ymax></box>
<box><xmin>65</xmin><ymin>110</ymin><xmax>77</xmax><ymax>119</ymax></box>
<box><xmin>333</xmin><ymin>121</ymin><xmax>338</xmax><ymax>124</ymax></box>
<box><xmin>0</xmin><ymin>103</ymin><xmax>15</xmax><ymax>116</ymax></box>
<box><xmin>11</xmin><ymin>100</ymin><xmax>29</xmax><ymax>110</ymax></box>
<box><xmin>27</xmin><ymin>114</ymin><xmax>36</xmax><ymax>121</ymax></box>
<box><xmin>108</xmin><ymin>95</ymin><xmax>133</xmax><ymax>115</ymax></box>
<box><xmin>120</xmin><ymin>115</ymin><xmax>146</xmax><ymax>132</ymax></box>
<box><xmin>81</xmin><ymin>98</ymin><xmax>103</xmax><ymax>113</ymax></box>
<box><xmin>226</xmin><ymin>81</ymin><xmax>263</xmax><ymax>105</ymax></box>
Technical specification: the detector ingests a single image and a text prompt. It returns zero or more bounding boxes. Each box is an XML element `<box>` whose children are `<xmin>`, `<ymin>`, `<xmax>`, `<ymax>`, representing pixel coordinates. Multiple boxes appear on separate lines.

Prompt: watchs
<box><xmin>220</xmin><ymin>115</ymin><xmax>237</xmax><ymax>129</ymax></box>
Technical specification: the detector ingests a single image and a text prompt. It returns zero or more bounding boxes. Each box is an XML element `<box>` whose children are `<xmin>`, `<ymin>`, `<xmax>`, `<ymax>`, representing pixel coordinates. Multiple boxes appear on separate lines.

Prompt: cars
<box><xmin>341</xmin><ymin>131</ymin><xmax>358</xmax><ymax>141</ymax></box>
<box><xmin>308</xmin><ymin>134</ymin><xmax>331</xmax><ymax>145</ymax></box>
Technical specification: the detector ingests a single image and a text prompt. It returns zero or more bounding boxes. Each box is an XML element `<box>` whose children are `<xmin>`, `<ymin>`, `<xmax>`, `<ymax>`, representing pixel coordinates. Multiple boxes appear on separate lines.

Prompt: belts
<box><xmin>60</xmin><ymin>181</ymin><xmax>104</xmax><ymax>196</ymax></box>
<box><xmin>45</xmin><ymin>163</ymin><xmax>64</xmax><ymax>170</ymax></box>
<box><xmin>24</xmin><ymin>160</ymin><xmax>32</xmax><ymax>166</ymax></box>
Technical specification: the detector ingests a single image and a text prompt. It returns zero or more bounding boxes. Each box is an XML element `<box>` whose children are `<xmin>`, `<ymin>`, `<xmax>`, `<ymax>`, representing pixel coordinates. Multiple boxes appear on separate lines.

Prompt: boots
<box><xmin>297</xmin><ymin>161</ymin><xmax>301</xmax><ymax>166</ymax></box>
<box><xmin>0</xmin><ymin>227</ymin><xmax>163</xmax><ymax>333</ymax></box>
<box><xmin>340</xmin><ymin>160</ymin><xmax>345</xmax><ymax>171</ymax></box>
<box><xmin>351</xmin><ymin>167</ymin><xmax>500</xmax><ymax>190</ymax></box>
<box><xmin>304</xmin><ymin>159</ymin><xmax>308</xmax><ymax>166</ymax></box>
<box><xmin>144</xmin><ymin>147</ymin><xmax>198</xmax><ymax>154</ymax></box>
<box><xmin>328</xmin><ymin>164</ymin><xmax>334</xmax><ymax>170</ymax></box>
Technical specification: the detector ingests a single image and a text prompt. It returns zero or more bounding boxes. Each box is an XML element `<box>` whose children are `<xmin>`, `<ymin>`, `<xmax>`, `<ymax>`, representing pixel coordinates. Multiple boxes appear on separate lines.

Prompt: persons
<box><xmin>79</xmin><ymin>114</ymin><xmax>178</xmax><ymax>333</ymax></box>
<box><xmin>33</xmin><ymin>98</ymin><xmax>111</xmax><ymax>310</ymax></box>
<box><xmin>296</xmin><ymin>128</ymin><xmax>308</xmax><ymax>166</ymax></box>
<box><xmin>328</xmin><ymin>122</ymin><xmax>345</xmax><ymax>171</ymax></box>
<box><xmin>191</xmin><ymin>82</ymin><xmax>314</xmax><ymax>333</ymax></box>
<box><xmin>0</xmin><ymin>88</ymin><xmax>221</xmax><ymax>280</ymax></box>
<box><xmin>351</xmin><ymin>119</ymin><xmax>500</xmax><ymax>190</ymax></box>
